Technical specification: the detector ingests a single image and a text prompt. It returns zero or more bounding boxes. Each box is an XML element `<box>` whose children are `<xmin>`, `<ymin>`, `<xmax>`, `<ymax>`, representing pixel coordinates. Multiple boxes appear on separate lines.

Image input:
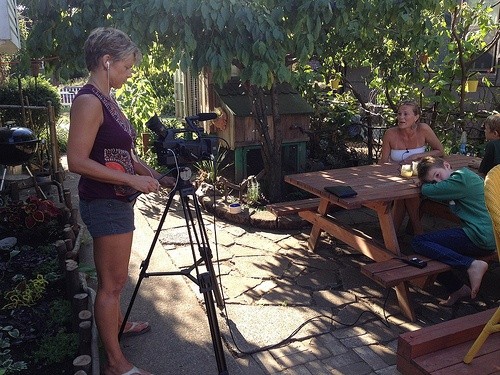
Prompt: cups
<box><xmin>402</xmin><ymin>162</ymin><xmax>418</xmax><ymax>178</ymax></box>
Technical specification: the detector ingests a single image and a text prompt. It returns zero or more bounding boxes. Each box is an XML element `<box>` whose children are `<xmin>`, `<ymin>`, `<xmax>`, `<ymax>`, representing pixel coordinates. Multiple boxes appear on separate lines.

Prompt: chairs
<box><xmin>463</xmin><ymin>164</ymin><xmax>500</xmax><ymax>364</ymax></box>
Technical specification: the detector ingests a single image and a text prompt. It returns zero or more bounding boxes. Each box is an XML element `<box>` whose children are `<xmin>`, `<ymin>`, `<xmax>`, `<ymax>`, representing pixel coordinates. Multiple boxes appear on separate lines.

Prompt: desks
<box><xmin>284</xmin><ymin>154</ymin><xmax>489</xmax><ymax>323</ymax></box>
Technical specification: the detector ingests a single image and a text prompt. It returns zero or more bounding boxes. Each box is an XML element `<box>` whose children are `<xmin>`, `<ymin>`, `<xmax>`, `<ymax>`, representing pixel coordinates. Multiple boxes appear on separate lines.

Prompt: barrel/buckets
<box><xmin>330</xmin><ymin>75</ymin><xmax>338</xmax><ymax>89</ymax></box>
<box><xmin>466</xmin><ymin>72</ymin><xmax>478</xmax><ymax>93</ymax></box>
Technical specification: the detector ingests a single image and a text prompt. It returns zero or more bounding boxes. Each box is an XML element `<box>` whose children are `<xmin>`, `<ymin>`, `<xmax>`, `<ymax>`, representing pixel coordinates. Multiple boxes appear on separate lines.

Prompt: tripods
<box><xmin>119</xmin><ymin>167</ymin><xmax>229</xmax><ymax>375</ymax></box>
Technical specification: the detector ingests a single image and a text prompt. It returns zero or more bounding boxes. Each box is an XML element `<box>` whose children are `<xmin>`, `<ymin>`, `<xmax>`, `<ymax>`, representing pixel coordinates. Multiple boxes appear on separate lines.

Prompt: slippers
<box><xmin>109</xmin><ymin>366</ymin><xmax>151</xmax><ymax>375</ymax></box>
<box><xmin>119</xmin><ymin>322</ymin><xmax>150</xmax><ymax>337</ymax></box>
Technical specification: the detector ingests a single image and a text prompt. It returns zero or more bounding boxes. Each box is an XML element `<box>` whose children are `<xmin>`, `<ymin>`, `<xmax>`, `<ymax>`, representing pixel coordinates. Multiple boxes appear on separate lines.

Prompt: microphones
<box><xmin>406</xmin><ymin>148</ymin><xmax>409</xmax><ymax>153</ymax></box>
<box><xmin>194</xmin><ymin>113</ymin><xmax>217</xmax><ymax>121</ymax></box>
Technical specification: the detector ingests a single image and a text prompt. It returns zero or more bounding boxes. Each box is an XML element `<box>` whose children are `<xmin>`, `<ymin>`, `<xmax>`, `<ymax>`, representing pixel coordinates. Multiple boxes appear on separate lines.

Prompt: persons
<box><xmin>379</xmin><ymin>101</ymin><xmax>445</xmax><ymax>237</ymax></box>
<box><xmin>66</xmin><ymin>28</ymin><xmax>176</xmax><ymax>375</ymax></box>
<box><xmin>468</xmin><ymin>113</ymin><xmax>500</xmax><ymax>174</ymax></box>
<box><xmin>409</xmin><ymin>155</ymin><xmax>497</xmax><ymax>306</ymax></box>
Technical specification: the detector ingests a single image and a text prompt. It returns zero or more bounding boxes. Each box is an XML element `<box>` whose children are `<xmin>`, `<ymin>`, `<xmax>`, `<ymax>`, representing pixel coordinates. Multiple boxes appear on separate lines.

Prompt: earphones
<box><xmin>106</xmin><ymin>61</ymin><xmax>109</xmax><ymax>68</ymax></box>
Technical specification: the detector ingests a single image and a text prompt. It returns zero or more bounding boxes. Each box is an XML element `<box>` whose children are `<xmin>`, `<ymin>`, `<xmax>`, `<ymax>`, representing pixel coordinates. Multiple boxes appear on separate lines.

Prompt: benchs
<box><xmin>266</xmin><ymin>197</ymin><xmax>340</xmax><ymax>224</ymax></box>
<box><xmin>360</xmin><ymin>251</ymin><xmax>500</xmax><ymax>289</ymax></box>
